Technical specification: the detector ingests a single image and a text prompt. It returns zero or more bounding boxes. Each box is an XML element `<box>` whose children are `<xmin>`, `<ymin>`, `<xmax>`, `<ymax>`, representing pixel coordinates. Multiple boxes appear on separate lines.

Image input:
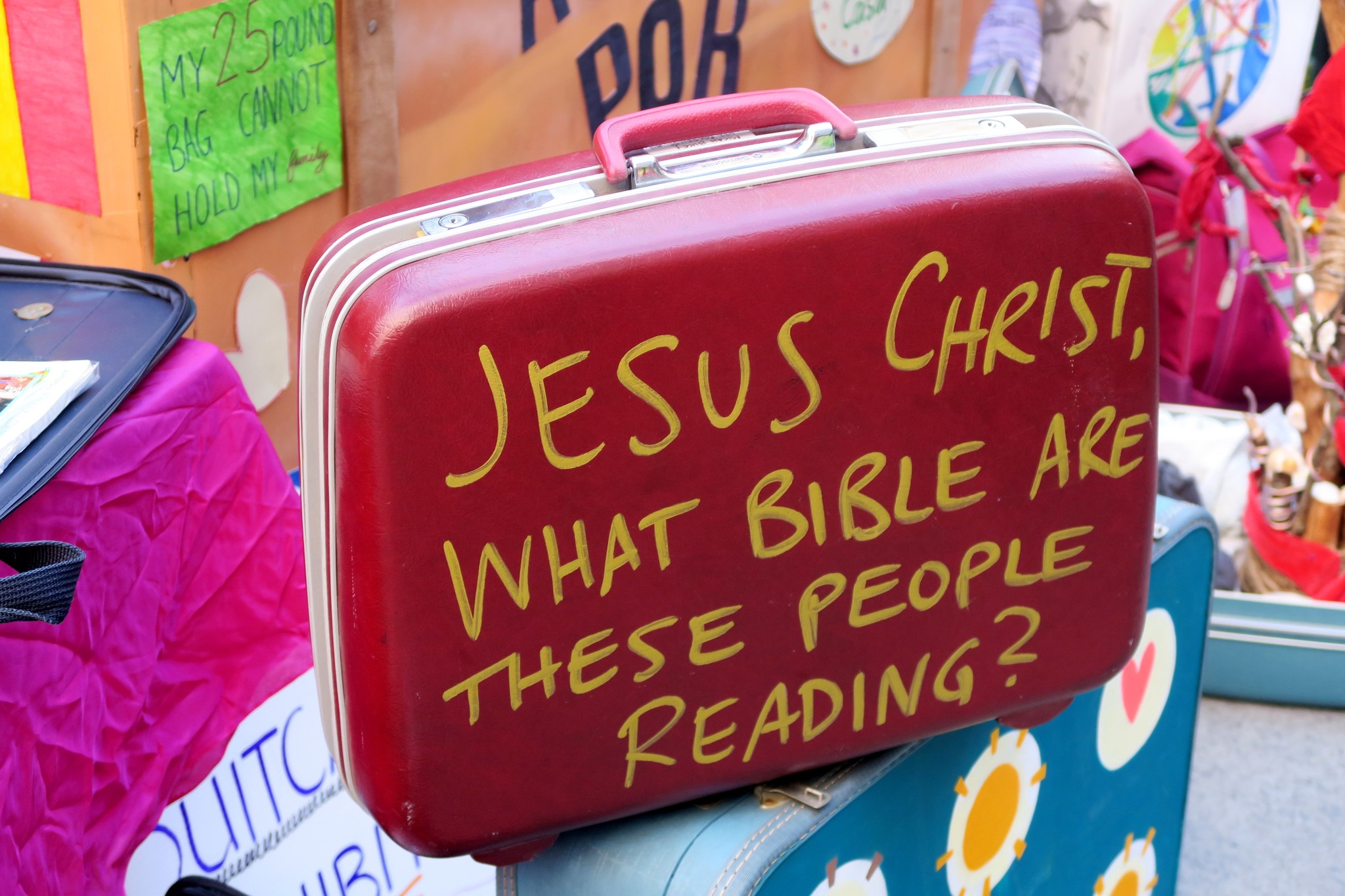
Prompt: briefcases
<box><xmin>495</xmin><ymin>491</ymin><xmax>1219</xmax><ymax>896</ymax></box>
<box><xmin>294</xmin><ymin>88</ymin><xmax>1158</xmax><ymax>868</ymax></box>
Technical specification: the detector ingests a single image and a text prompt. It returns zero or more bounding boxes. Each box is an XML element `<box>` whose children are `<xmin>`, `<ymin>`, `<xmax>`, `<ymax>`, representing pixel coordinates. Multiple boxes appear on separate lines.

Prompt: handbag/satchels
<box><xmin>1116</xmin><ymin>124</ymin><xmax>1319</xmax><ymax>412</ymax></box>
